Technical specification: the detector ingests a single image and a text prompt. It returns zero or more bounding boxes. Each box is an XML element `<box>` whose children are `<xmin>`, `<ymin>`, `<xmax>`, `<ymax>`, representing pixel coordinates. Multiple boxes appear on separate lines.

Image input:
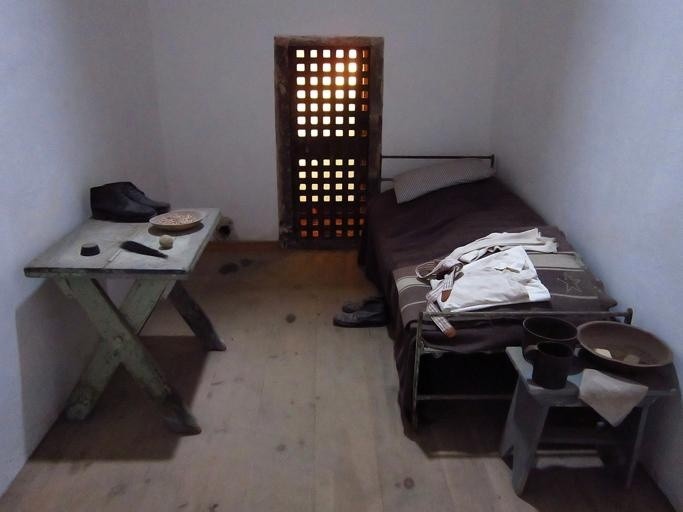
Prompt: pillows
<box><xmin>392</xmin><ymin>155</ymin><xmax>497</xmax><ymax>205</ymax></box>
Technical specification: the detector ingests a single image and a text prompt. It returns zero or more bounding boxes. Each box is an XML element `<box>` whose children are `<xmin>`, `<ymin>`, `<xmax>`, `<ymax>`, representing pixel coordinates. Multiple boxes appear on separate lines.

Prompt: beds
<box><xmin>364</xmin><ymin>150</ymin><xmax>634</xmax><ymax>436</ymax></box>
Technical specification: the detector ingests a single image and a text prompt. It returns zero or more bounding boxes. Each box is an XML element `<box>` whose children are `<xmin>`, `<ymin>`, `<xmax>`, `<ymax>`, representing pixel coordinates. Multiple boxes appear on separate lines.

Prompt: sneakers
<box><xmin>334</xmin><ymin>310</ymin><xmax>387</xmax><ymax>327</ymax></box>
<box><xmin>122</xmin><ymin>181</ymin><xmax>169</xmax><ymax>213</ymax></box>
<box><xmin>90</xmin><ymin>183</ymin><xmax>155</xmax><ymax>223</ymax></box>
<box><xmin>342</xmin><ymin>296</ymin><xmax>393</xmax><ymax>313</ymax></box>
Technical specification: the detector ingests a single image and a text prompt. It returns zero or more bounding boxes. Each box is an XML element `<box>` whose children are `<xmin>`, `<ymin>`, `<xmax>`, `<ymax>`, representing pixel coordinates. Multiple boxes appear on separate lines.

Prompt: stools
<box><xmin>499</xmin><ymin>344</ymin><xmax>678</xmax><ymax>498</ymax></box>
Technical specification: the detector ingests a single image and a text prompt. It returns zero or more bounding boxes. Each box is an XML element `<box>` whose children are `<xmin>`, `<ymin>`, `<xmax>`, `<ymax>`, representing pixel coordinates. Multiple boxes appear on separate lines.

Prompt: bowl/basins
<box><xmin>520</xmin><ymin>315</ymin><xmax>578</xmax><ymax>347</ymax></box>
<box><xmin>577</xmin><ymin>321</ymin><xmax>675</xmax><ymax>373</ymax></box>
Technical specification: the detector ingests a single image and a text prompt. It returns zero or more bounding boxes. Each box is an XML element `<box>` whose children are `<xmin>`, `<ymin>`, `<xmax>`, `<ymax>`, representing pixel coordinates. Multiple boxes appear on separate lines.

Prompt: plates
<box><xmin>148</xmin><ymin>209</ymin><xmax>211</xmax><ymax>233</ymax></box>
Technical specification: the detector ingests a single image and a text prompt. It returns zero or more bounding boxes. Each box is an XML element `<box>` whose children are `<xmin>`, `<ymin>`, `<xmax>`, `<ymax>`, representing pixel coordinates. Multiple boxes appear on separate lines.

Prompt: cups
<box><xmin>524</xmin><ymin>342</ymin><xmax>574</xmax><ymax>390</ymax></box>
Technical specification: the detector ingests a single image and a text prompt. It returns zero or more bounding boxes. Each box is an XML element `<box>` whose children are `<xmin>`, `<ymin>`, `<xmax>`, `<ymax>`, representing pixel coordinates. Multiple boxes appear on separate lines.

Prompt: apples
<box><xmin>159</xmin><ymin>235</ymin><xmax>173</xmax><ymax>249</ymax></box>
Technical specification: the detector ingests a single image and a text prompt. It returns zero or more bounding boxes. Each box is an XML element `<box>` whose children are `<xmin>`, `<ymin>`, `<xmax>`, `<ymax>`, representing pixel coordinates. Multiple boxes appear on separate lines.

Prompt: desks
<box><xmin>22</xmin><ymin>203</ymin><xmax>229</xmax><ymax>438</ymax></box>
